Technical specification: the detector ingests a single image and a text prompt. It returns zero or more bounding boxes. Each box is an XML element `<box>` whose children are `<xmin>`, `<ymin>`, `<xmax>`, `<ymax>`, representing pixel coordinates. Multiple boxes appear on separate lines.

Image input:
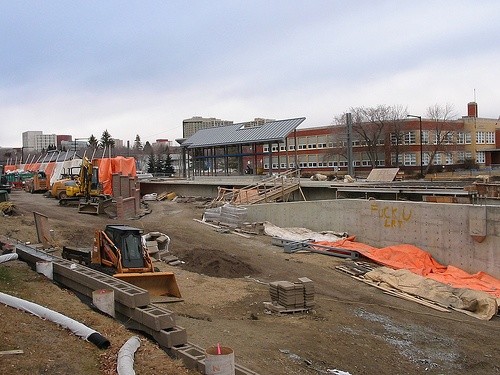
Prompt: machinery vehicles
<box><xmin>0</xmin><ymin>154</ymin><xmax>121</xmax><ymax>219</ymax></box>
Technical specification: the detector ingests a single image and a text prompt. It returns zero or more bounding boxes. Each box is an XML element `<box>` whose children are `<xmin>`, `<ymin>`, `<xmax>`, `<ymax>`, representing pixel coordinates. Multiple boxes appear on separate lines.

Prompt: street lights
<box><xmin>407</xmin><ymin>114</ymin><xmax>425</xmax><ymax>178</ymax></box>
